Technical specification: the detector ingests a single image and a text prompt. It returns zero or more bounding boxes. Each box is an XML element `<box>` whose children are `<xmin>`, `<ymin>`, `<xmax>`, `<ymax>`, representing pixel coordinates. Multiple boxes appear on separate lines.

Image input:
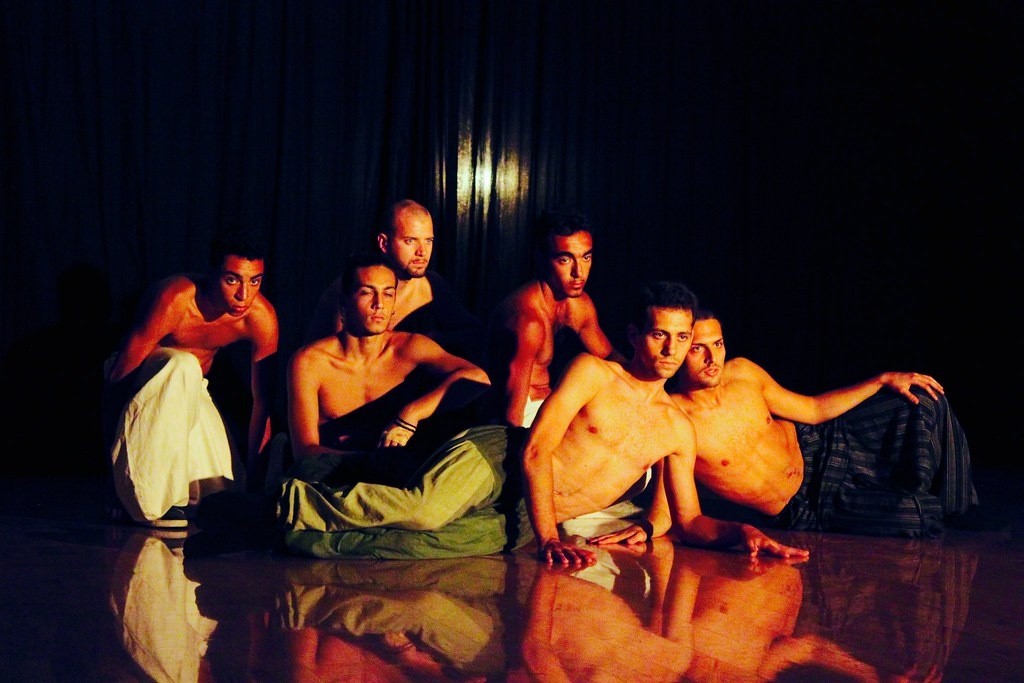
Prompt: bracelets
<box><xmin>391</xmin><ymin>643</ymin><xmax>414</xmax><ymax>653</ymax></box>
<box><xmin>394</xmin><ymin>416</ymin><xmax>418</xmax><ymax>434</ymax></box>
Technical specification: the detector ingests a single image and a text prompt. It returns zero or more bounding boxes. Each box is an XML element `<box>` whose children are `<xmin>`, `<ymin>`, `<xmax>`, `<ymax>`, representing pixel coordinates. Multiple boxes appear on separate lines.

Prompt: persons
<box><xmin>585</xmin><ymin>308</ymin><xmax>1011</xmax><ymax>544</ymax></box>
<box><xmin>123</xmin><ymin>542</ymin><xmax>877</xmax><ymax>683</ymax></box>
<box><xmin>283</xmin><ymin>256</ymin><xmax>492</xmax><ymax>485</ymax></box>
<box><xmin>325</xmin><ymin>199</ymin><xmax>435</xmax><ymax>330</ymax></box>
<box><xmin>485</xmin><ymin>218</ymin><xmax>632</xmax><ymax>428</ymax></box>
<box><xmin>184</xmin><ymin>280</ymin><xmax>809</xmax><ymax>564</ymax></box>
<box><xmin>113</xmin><ymin>226</ymin><xmax>280</xmax><ymax>527</ymax></box>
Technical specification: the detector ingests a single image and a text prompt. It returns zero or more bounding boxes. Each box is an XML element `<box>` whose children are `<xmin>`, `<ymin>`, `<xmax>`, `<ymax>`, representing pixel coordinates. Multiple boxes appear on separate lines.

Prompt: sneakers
<box><xmin>149</xmin><ymin>531</ymin><xmax>188</xmax><ymax>550</ymax></box>
<box><xmin>138</xmin><ymin>506</ymin><xmax>190</xmax><ymax>529</ymax></box>
